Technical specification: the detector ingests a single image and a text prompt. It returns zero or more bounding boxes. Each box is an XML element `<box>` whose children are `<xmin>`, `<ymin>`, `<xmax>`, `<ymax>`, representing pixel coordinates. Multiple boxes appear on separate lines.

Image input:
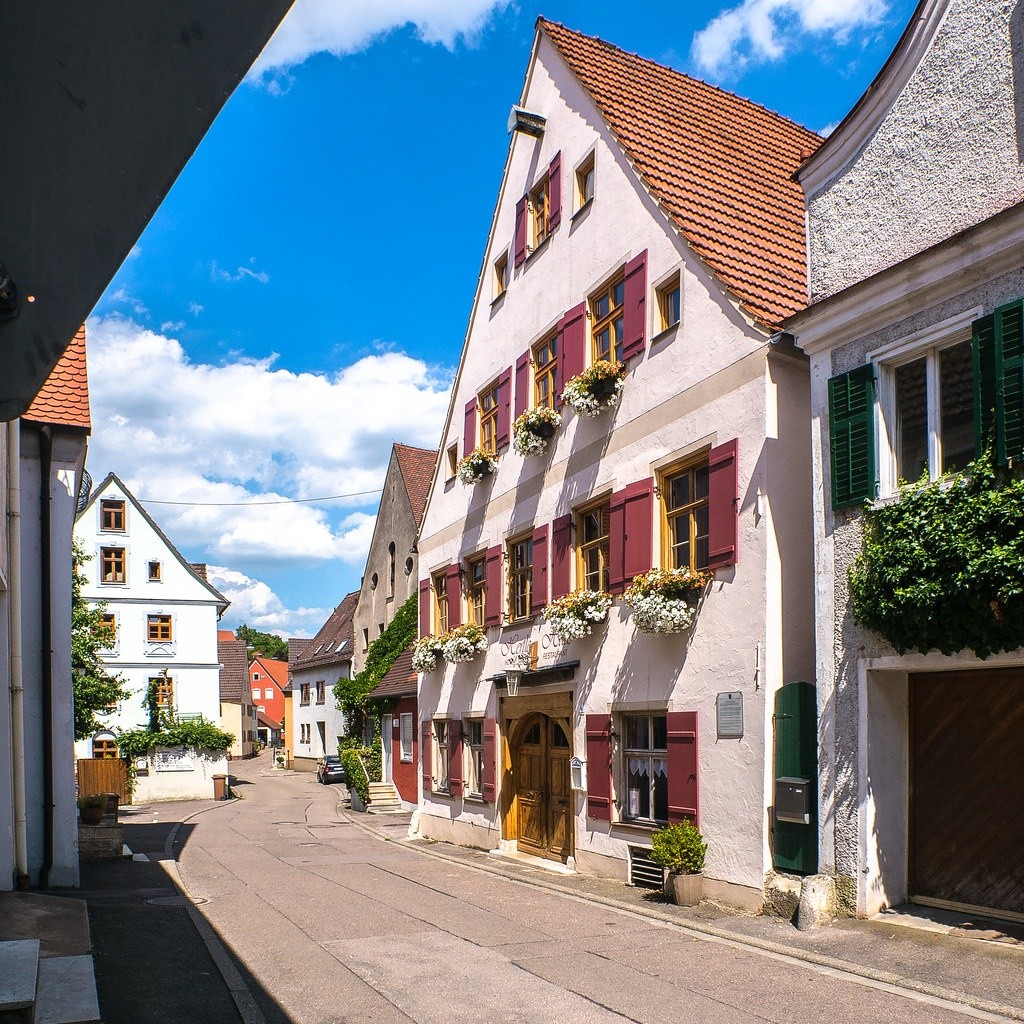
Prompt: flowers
<box><xmin>455</xmin><ymin>446</ymin><xmax>500</xmax><ymax>486</ymax></box>
<box><xmin>540</xmin><ymin>588</ymin><xmax>614</xmax><ymax>641</ymax></box>
<box><xmin>439</xmin><ymin>624</ymin><xmax>490</xmax><ymax>664</ymax></box>
<box><xmin>512</xmin><ymin>406</ymin><xmax>564</xmax><ymax>457</ymax></box>
<box><xmin>559</xmin><ymin>359</ymin><xmax>626</xmax><ymax>419</ymax></box>
<box><xmin>411</xmin><ymin>634</ymin><xmax>444</xmax><ymax>675</ymax></box>
<box><xmin>616</xmin><ymin>563</ymin><xmax>716</xmax><ymax>637</ymax></box>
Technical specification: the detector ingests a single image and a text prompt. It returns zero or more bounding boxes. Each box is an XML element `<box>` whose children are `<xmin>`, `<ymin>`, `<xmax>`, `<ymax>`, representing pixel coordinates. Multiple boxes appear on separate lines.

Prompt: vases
<box><xmin>528</xmin><ymin>420</ymin><xmax>554</xmax><ymax>438</ymax></box>
<box><xmin>473</xmin><ymin>460</ymin><xmax>493</xmax><ymax>475</ymax></box>
<box><xmin>670</xmin><ymin>585</ymin><xmax>701</xmax><ymax>605</ymax></box>
<box><xmin>587</xmin><ymin>374</ymin><xmax>616</xmax><ymax>402</ymax></box>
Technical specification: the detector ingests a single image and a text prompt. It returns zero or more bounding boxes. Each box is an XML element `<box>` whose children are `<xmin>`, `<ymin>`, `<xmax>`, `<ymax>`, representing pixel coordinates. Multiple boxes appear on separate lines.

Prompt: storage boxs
<box><xmin>775</xmin><ymin>776</ymin><xmax>814</xmax><ymax>825</ymax></box>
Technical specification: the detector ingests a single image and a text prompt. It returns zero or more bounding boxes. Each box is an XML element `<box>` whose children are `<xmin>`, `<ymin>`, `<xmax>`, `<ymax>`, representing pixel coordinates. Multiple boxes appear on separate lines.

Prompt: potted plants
<box><xmin>276</xmin><ymin>757</ymin><xmax>285</xmax><ymax>769</ymax></box>
<box><xmin>646</xmin><ymin>816</ymin><xmax>709</xmax><ymax>907</ymax></box>
<box><xmin>76</xmin><ymin>793</ymin><xmax>109</xmax><ymax>826</ymax></box>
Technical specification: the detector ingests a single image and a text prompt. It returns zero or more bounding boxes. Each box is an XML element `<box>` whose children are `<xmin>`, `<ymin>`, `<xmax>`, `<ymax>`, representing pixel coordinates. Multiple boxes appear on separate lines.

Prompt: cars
<box><xmin>317</xmin><ymin>754</ymin><xmax>347</xmax><ymax>785</ymax></box>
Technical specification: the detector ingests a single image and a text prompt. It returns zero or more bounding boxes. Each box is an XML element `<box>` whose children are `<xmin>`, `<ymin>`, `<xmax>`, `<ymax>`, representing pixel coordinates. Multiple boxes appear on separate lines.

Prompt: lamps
<box><xmin>501</xmin><ymin>655</ymin><xmax>539</xmax><ymax>697</ymax></box>
